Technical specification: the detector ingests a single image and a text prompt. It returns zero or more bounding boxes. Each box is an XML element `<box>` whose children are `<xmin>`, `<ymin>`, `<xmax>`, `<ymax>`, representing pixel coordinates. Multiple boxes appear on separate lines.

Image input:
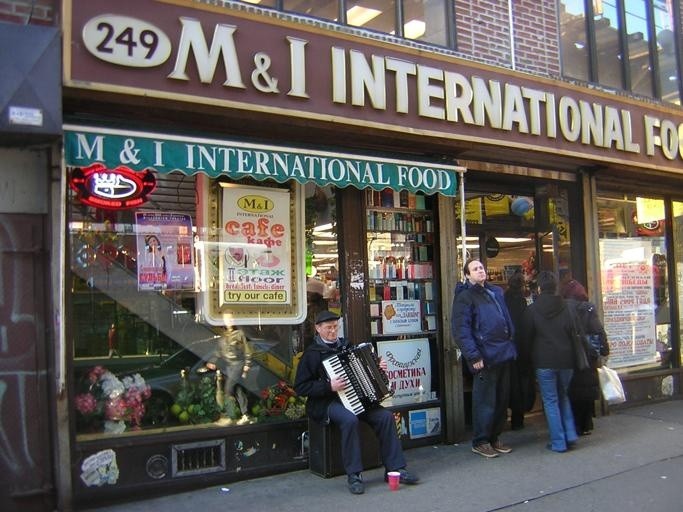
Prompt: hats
<box><xmin>316</xmin><ymin>311</ymin><xmax>340</xmax><ymax>325</ymax></box>
<box><xmin>562</xmin><ymin>280</ymin><xmax>586</xmax><ymax>296</ymax></box>
<box><xmin>509</xmin><ymin>274</ymin><xmax>526</xmax><ymax>287</ymax></box>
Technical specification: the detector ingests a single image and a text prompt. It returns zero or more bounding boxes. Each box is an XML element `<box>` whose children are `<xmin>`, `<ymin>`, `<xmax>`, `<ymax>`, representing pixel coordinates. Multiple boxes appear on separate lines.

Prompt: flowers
<box><xmin>251</xmin><ymin>379</ymin><xmax>308</xmax><ymax>420</ymax></box>
<box><xmin>73</xmin><ymin>366</ymin><xmax>152</xmax><ymax>430</ymax></box>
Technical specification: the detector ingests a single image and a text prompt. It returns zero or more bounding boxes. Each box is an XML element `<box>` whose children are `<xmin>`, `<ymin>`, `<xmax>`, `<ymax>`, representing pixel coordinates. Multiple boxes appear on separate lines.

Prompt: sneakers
<box><xmin>348</xmin><ymin>472</ymin><xmax>364</xmax><ymax>493</ymax></box>
<box><xmin>492</xmin><ymin>439</ymin><xmax>513</xmax><ymax>453</ymax></box>
<box><xmin>547</xmin><ymin>442</ymin><xmax>552</xmax><ymax>449</ymax></box>
<box><xmin>512</xmin><ymin>422</ymin><xmax>524</xmax><ymax>430</ymax></box>
<box><xmin>577</xmin><ymin>427</ymin><xmax>591</xmax><ymax>435</ymax></box>
<box><xmin>384</xmin><ymin>467</ymin><xmax>419</xmax><ymax>483</ymax></box>
<box><xmin>471</xmin><ymin>443</ymin><xmax>497</xmax><ymax>457</ymax></box>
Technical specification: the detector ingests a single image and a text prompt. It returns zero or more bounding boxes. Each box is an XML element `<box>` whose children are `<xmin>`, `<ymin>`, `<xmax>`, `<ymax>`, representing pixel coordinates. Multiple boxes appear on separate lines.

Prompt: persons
<box><xmin>292</xmin><ymin>311</ymin><xmax>420</xmax><ymax>494</ymax></box>
<box><xmin>145</xmin><ymin>235</ymin><xmax>166</xmax><ymax>276</ymax></box>
<box><xmin>107</xmin><ymin>322</ymin><xmax>122</xmax><ymax>360</ymax></box>
<box><xmin>206</xmin><ymin>307</ymin><xmax>252</xmax><ymax>426</ymax></box>
<box><xmin>301</xmin><ymin>279</ymin><xmax>328</xmax><ymax>339</ymax></box>
<box><xmin>450</xmin><ymin>259</ymin><xmax>610</xmax><ymax>458</ymax></box>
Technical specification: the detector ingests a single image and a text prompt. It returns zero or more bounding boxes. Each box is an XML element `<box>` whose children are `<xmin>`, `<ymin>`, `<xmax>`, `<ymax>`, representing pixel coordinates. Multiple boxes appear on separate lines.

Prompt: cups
<box><xmin>387</xmin><ymin>472</ymin><xmax>401</xmax><ymax>491</ymax></box>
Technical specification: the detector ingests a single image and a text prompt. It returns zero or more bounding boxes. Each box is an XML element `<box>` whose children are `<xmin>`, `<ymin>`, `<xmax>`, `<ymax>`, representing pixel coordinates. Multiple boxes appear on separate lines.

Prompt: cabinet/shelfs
<box><xmin>490</xmin><ymin>280</ymin><xmax>507</xmax><ymax>285</ymax></box>
<box><xmin>366</xmin><ymin>205</ymin><xmax>437</xmax><ymax>336</ymax></box>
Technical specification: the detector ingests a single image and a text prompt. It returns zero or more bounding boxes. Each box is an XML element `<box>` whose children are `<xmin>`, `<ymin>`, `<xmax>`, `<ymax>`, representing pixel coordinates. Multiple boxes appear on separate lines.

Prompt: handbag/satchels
<box><xmin>565</xmin><ymin>331</ymin><xmax>598</xmax><ymax>366</ymax></box>
<box><xmin>596</xmin><ymin>365</ymin><xmax>626</xmax><ymax>405</ymax></box>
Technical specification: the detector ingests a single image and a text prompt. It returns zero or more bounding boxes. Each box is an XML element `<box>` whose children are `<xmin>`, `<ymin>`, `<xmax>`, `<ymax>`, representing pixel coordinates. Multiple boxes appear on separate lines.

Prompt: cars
<box><xmin>87</xmin><ymin>333</ymin><xmax>282</xmax><ymax>429</ymax></box>
